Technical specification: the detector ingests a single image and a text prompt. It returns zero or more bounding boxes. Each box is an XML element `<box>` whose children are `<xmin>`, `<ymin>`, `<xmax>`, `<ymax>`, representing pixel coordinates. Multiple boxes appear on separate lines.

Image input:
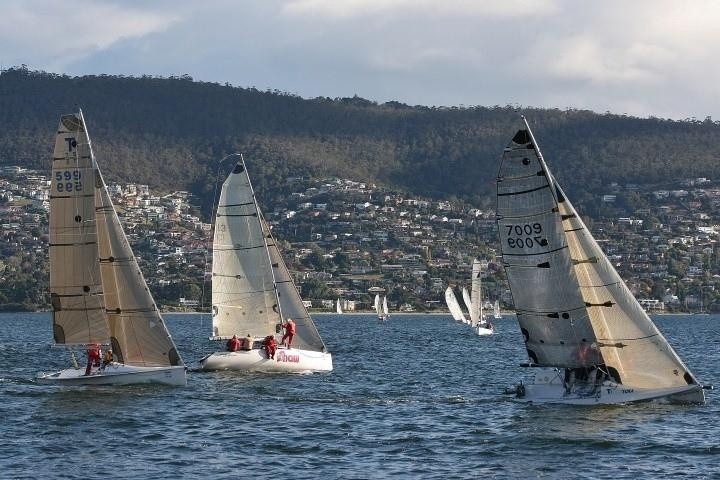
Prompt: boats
<box><xmin>494</xmin><ymin>110</ymin><xmax>714</xmax><ymax>410</ymax></box>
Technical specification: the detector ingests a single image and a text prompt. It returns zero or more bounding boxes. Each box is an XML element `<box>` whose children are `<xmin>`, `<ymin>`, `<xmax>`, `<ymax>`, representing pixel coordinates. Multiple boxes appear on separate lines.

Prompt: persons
<box><xmin>97</xmin><ymin>349</ymin><xmax>112</xmax><ymax>371</ymax></box>
<box><xmin>226</xmin><ymin>335</ymin><xmax>240</xmax><ymax>352</ymax></box>
<box><xmin>83</xmin><ymin>342</ymin><xmax>103</xmax><ymax>376</ymax></box>
<box><xmin>264</xmin><ymin>335</ymin><xmax>278</xmax><ymax>359</ymax></box>
<box><xmin>241</xmin><ymin>334</ymin><xmax>254</xmax><ymax>351</ymax></box>
<box><xmin>279</xmin><ymin>318</ymin><xmax>296</xmax><ymax>349</ymax></box>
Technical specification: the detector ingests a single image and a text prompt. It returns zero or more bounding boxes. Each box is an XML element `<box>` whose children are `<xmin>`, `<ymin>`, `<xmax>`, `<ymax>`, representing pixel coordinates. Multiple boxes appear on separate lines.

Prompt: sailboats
<box><xmin>36</xmin><ymin>105</ymin><xmax>193</xmax><ymax>390</ymax></box>
<box><xmin>381</xmin><ymin>295</ymin><xmax>391</xmax><ymax>317</ymax></box>
<box><xmin>336</xmin><ymin>297</ymin><xmax>343</xmax><ymax>315</ymax></box>
<box><xmin>187</xmin><ymin>147</ymin><xmax>341</xmax><ymax>375</ymax></box>
<box><xmin>444</xmin><ymin>258</ymin><xmax>506</xmax><ymax>338</ymax></box>
<box><xmin>372</xmin><ymin>293</ymin><xmax>388</xmax><ymax>320</ymax></box>
